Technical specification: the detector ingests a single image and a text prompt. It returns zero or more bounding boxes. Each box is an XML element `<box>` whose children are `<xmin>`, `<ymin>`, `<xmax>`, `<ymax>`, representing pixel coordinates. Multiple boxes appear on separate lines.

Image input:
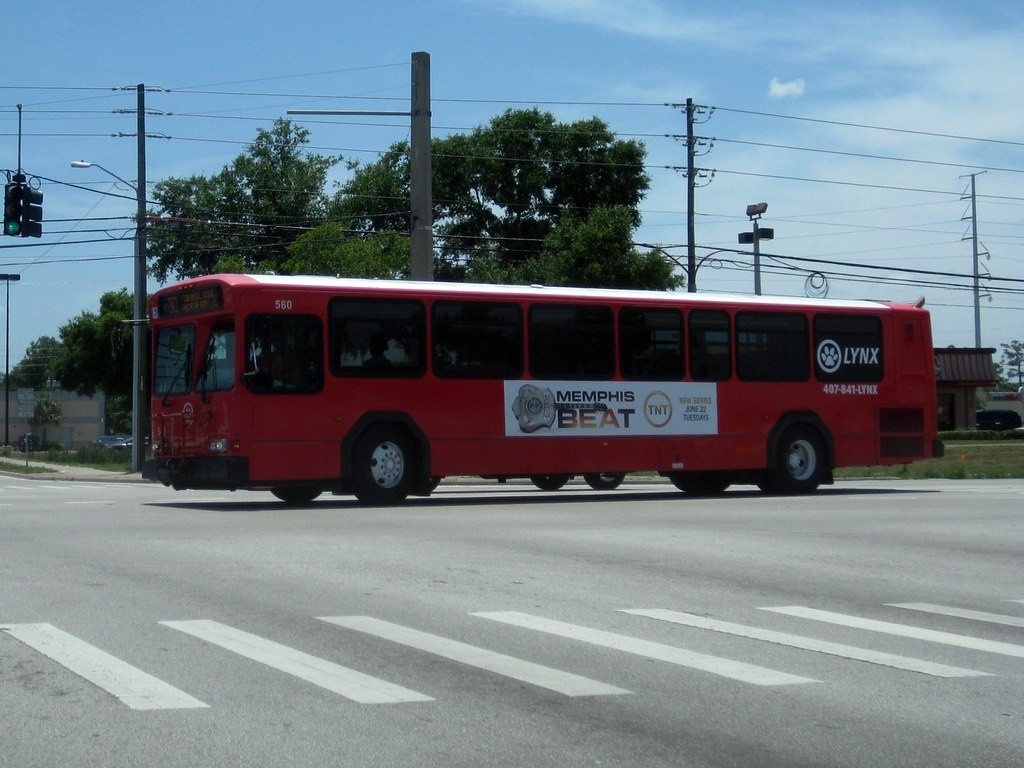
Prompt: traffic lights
<box><xmin>4</xmin><ymin>184</ymin><xmax>23</xmax><ymax>236</ymax></box>
<box><xmin>21</xmin><ymin>187</ymin><xmax>43</xmax><ymax>237</ymax></box>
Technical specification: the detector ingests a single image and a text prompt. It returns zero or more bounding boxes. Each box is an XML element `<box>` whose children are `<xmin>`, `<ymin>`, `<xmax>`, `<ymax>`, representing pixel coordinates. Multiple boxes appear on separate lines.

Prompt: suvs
<box><xmin>17</xmin><ymin>432</ymin><xmax>42</xmax><ymax>452</ymax></box>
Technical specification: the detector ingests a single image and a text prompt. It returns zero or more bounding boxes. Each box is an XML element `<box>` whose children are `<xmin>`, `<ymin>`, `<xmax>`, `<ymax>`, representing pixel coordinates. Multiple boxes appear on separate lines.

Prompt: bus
<box><xmin>145</xmin><ymin>271</ymin><xmax>943</xmax><ymax>505</ymax></box>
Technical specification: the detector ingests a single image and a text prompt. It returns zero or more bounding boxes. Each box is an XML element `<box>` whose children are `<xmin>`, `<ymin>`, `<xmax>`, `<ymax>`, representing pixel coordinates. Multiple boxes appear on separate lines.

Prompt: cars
<box><xmin>92</xmin><ymin>435</ymin><xmax>125</xmax><ymax>451</ymax></box>
<box><xmin>105</xmin><ymin>436</ymin><xmax>134</xmax><ymax>452</ymax></box>
<box><xmin>976</xmin><ymin>410</ymin><xmax>1023</xmax><ymax>432</ymax></box>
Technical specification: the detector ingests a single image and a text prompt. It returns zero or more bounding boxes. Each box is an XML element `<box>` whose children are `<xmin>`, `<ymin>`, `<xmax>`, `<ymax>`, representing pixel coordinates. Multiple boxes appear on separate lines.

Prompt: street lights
<box><xmin>736</xmin><ymin>202</ymin><xmax>775</xmax><ymax>295</ymax></box>
<box><xmin>70</xmin><ymin>160</ymin><xmax>139</xmax><ymax>193</ymax></box>
